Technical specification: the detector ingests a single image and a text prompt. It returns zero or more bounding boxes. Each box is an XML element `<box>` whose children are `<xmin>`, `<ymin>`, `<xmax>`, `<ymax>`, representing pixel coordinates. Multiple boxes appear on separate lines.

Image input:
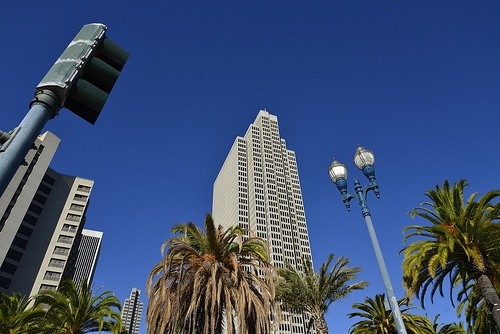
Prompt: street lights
<box><xmin>329</xmin><ymin>143</ymin><xmax>408</xmax><ymax>334</ymax></box>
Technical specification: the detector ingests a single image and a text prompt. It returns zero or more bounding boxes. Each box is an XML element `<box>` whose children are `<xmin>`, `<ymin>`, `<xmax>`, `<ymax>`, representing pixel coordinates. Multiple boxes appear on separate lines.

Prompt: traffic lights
<box><xmin>34</xmin><ymin>23</ymin><xmax>130</xmax><ymax>125</ymax></box>
